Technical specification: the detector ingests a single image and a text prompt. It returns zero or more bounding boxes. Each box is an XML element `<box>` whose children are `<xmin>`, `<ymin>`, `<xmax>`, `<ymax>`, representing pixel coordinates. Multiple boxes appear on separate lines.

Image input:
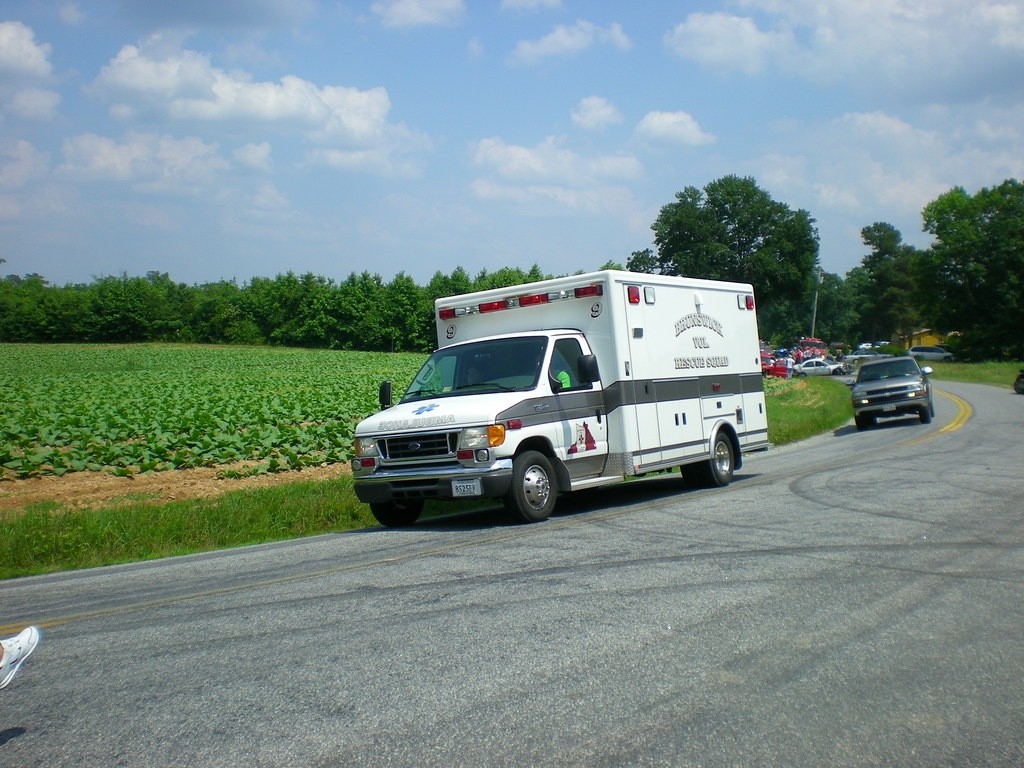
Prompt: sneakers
<box><xmin>0</xmin><ymin>626</ymin><xmax>39</xmax><ymax>689</ymax></box>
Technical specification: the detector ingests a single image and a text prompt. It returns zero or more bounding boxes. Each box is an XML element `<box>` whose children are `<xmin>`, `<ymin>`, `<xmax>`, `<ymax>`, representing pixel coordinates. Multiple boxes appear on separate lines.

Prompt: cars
<box><xmin>760</xmin><ymin>335</ymin><xmax>890</xmax><ymax>377</ymax></box>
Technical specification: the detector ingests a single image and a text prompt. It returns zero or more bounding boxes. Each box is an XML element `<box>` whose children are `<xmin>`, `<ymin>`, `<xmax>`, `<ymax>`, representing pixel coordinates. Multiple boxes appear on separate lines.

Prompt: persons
<box><xmin>536</xmin><ymin>355</ymin><xmax>570</xmax><ymax>390</ymax></box>
<box><xmin>0</xmin><ymin>625</ymin><xmax>39</xmax><ymax>690</ymax></box>
<box><xmin>778</xmin><ymin>346</ymin><xmax>845</xmax><ymax>381</ymax></box>
<box><xmin>904</xmin><ymin>367</ymin><xmax>914</xmax><ymax>376</ymax></box>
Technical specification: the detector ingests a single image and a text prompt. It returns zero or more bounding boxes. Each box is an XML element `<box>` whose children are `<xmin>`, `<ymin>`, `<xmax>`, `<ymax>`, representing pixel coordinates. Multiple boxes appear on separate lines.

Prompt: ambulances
<box><xmin>349</xmin><ymin>267</ymin><xmax>773</xmax><ymax>528</ymax></box>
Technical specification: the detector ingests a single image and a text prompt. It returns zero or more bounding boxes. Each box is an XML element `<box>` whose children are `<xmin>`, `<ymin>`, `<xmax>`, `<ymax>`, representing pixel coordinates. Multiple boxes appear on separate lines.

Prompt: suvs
<box><xmin>905</xmin><ymin>346</ymin><xmax>955</xmax><ymax>362</ymax></box>
<box><xmin>845</xmin><ymin>356</ymin><xmax>936</xmax><ymax>431</ymax></box>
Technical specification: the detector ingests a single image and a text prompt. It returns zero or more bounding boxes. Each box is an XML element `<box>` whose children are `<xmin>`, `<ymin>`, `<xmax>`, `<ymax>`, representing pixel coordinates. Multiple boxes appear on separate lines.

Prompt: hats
<box><xmin>789</xmin><ymin>355</ymin><xmax>792</xmax><ymax>358</ymax></box>
